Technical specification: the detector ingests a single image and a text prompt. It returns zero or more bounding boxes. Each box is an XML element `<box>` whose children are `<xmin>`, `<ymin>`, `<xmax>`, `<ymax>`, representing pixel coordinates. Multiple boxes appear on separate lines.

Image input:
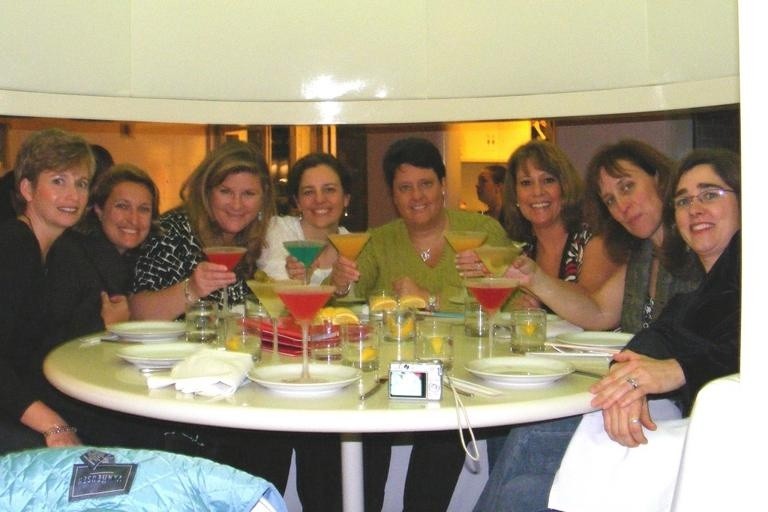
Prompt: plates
<box><xmin>556</xmin><ymin>329</ymin><xmax>634</xmax><ymax>349</ymax></box>
<box><xmin>245</xmin><ymin>363</ymin><xmax>363</xmax><ymax>399</ymax></box>
<box><xmin>114</xmin><ymin>342</ymin><xmax>211</xmax><ymax>367</ymax></box>
<box><xmin>105</xmin><ymin>319</ymin><xmax>187</xmax><ymax>339</ymax></box>
<box><xmin>465</xmin><ymin>359</ymin><xmax>574</xmax><ymax>387</ymax></box>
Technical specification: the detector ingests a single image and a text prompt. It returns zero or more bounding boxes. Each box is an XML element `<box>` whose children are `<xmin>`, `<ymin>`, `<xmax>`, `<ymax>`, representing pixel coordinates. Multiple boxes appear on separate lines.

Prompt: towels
<box><xmin>173</xmin><ymin>349</ymin><xmax>253</xmax><ymax>398</ymax></box>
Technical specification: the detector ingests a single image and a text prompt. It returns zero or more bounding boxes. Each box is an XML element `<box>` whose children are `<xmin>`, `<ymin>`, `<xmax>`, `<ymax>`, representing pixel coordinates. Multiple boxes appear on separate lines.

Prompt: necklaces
<box><xmin>404</xmin><ymin>218</ymin><xmax>450</xmax><ymax>269</ymax></box>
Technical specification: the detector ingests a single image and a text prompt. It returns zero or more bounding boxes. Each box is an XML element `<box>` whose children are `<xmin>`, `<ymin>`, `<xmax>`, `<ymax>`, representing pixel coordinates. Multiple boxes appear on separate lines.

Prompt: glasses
<box><xmin>669</xmin><ymin>189</ymin><xmax>739</xmax><ymax>209</ymax></box>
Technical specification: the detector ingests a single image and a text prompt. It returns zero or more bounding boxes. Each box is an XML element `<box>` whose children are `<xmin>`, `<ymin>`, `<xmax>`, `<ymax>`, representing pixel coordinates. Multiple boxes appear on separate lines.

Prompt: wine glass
<box><xmin>202</xmin><ymin>244</ymin><xmax>245</xmax><ymax>319</ymax></box>
<box><xmin>475</xmin><ymin>246</ymin><xmax>522</xmax><ymax>274</ymax></box>
<box><xmin>467</xmin><ymin>276</ymin><xmax>517</xmax><ymax>360</ymax></box>
<box><xmin>276</xmin><ymin>284</ymin><xmax>332</xmax><ymax>384</ymax></box>
<box><xmin>444</xmin><ymin>230</ymin><xmax>488</xmax><ymax>304</ymax></box>
<box><xmin>326</xmin><ymin>232</ymin><xmax>371</xmax><ymax>303</ymax></box>
<box><xmin>249</xmin><ymin>277</ymin><xmax>293</xmax><ymax>371</ymax></box>
<box><xmin>286</xmin><ymin>237</ymin><xmax>325</xmax><ymax>282</ymax></box>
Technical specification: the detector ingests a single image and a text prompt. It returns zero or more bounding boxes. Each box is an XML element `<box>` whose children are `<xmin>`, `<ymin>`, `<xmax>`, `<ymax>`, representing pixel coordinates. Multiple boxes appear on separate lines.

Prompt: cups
<box><xmin>223</xmin><ymin>312</ymin><xmax>260</xmax><ymax>362</ymax></box>
<box><xmin>181</xmin><ymin>299</ymin><xmax>218</xmax><ymax>342</ymax></box>
<box><xmin>460</xmin><ymin>295</ymin><xmax>490</xmax><ymax>336</ymax></box>
<box><xmin>417</xmin><ymin>322</ymin><xmax>456</xmax><ymax>373</ymax></box>
<box><xmin>513</xmin><ymin>308</ymin><xmax>547</xmax><ymax>354</ymax></box>
<box><xmin>342</xmin><ymin>316</ymin><xmax>381</xmax><ymax>374</ymax></box>
<box><xmin>383</xmin><ymin>303</ymin><xmax>415</xmax><ymax>344</ymax></box>
<box><xmin>309</xmin><ymin>318</ymin><xmax>344</xmax><ymax>358</ymax></box>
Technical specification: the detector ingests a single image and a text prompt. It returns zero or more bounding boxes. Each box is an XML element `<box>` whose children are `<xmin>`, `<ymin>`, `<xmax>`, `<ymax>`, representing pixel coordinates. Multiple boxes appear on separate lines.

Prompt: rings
<box><xmin>625</xmin><ymin>376</ymin><xmax>640</xmax><ymax>389</ymax></box>
<box><xmin>473</xmin><ymin>262</ymin><xmax>481</xmax><ymax>271</ymax></box>
<box><xmin>628</xmin><ymin>416</ymin><xmax>642</xmax><ymax>427</ymax></box>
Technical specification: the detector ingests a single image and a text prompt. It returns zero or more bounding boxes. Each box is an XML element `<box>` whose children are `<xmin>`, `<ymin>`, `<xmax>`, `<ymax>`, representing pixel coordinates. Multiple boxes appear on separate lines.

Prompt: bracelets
<box><xmin>38</xmin><ymin>424</ymin><xmax>84</xmax><ymax>440</ymax></box>
<box><xmin>179</xmin><ymin>278</ymin><xmax>201</xmax><ymax>309</ymax></box>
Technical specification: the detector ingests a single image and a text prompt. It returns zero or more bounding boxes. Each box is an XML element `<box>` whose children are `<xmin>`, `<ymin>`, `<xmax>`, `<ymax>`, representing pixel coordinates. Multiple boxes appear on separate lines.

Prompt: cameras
<box><xmin>388</xmin><ymin>358</ymin><xmax>444</xmax><ymax>401</ymax></box>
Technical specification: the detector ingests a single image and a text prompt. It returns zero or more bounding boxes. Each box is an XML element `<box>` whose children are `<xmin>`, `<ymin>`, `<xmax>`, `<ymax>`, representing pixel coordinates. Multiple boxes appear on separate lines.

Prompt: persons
<box><xmin>2</xmin><ymin>125</ymin><xmax>115</xmax><ymax>454</ymax></box>
<box><xmin>133</xmin><ymin>143</ymin><xmax>288</xmax><ymax>498</ymax></box>
<box><xmin>589</xmin><ymin>147</ymin><xmax>740</xmax><ymax>447</ymax></box>
<box><xmin>253</xmin><ymin>151</ymin><xmax>358</xmax><ymax>512</ymax></box>
<box><xmin>66</xmin><ymin>164</ymin><xmax>159</xmax><ymax>334</ymax></box>
<box><xmin>468</xmin><ymin>137</ymin><xmax>705</xmax><ymax>512</ymax></box>
<box><xmin>472</xmin><ymin>163</ymin><xmax>503</xmax><ymax>225</ymax></box>
<box><xmin>456</xmin><ymin>148</ymin><xmax>620</xmax><ymax>511</ymax></box>
<box><xmin>314</xmin><ymin>137</ymin><xmax>512</xmax><ymax>512</ymax></box>
